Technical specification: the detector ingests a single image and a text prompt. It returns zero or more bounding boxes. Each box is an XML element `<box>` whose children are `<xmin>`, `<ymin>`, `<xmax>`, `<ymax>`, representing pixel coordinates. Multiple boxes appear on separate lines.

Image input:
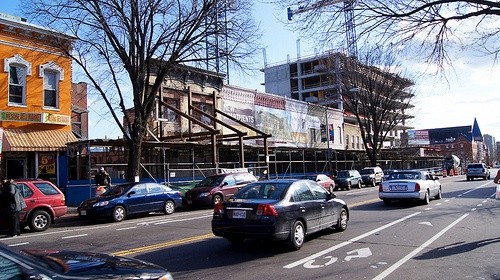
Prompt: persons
<box><xmin>94</xmin><ymin>167</ymin><xmax>112</xmax><ymax>190</ymax></box>
<box><xmin>0</xmin><ymin>177</ymin><xmax>28</xmax><ymax>238</ymax></box>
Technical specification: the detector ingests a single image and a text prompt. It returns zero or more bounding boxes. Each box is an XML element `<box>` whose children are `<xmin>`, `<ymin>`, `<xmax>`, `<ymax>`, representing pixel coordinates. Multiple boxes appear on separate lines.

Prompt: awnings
<box><xmin>2</xmin><ymin>128</ymin><xmax>78</xmax><ymax>151</ymax></box>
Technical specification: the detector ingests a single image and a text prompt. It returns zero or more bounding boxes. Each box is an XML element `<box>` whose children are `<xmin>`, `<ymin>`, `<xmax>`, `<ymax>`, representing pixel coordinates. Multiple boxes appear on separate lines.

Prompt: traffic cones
<box><xmin>449</xmin><ymin>168</ymin><xmax>454</xmax><ymax>177</ymax></box>
<box><xmin>442</xmin><ymin>169</ymin><xmax>447</xmax><ymax>178</ymax></box>
<box><xmin>455</xmin><ymin>168</ymin><xmax>459</xmax><ymax>176</ymax></box>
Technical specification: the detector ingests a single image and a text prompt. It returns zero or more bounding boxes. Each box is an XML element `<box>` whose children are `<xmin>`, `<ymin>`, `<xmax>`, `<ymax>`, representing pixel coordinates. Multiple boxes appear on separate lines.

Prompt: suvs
<box><xmin>0</xmin><ymin>177</ymin><xmax>69</xmax><ymax>237</ymax></box>
<box><xmin>465</xmin><ymin>162</ymin><xmax>491</xmax><ymax>182</ymax></box>
<box><xmin>332</xmin><ymin>169</ymin><xmax>364</xmax><ymax>191</ymax></box>
<box><xmin>359</xmin><ymin>166</ymin><xmax>384</xmax><ymax>187</ymax></box>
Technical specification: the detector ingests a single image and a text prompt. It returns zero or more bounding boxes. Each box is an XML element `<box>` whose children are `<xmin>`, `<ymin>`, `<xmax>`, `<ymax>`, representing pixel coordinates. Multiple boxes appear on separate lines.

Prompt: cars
<box><xmin>383</xmin><ymin>169</ymin><xmax>398</xmax><ymax>181</ymax></box>
<box><xmin>376</xmin><ymin>169</ymin><xmax>443</xmax><ymax>205</ymax></box>
<box><xmin>0</xmin><ymin>240</ymin><xmax>173</xmax><ymax>280</ymax></box>
<box><xmin>210</xmin><ymin>177</ymin><xmax>351</xmax><ymax>251</ymax></box>
<box><xmin>76</xmin><ymin>181</ymin><xmax>182</xmax><ymax>223</ymax></box>
<box><xmin>300</xmin><ymin>174</ymin><xmax>336</xmax><ymax>193</ymax></box>
<box><xmin>182</xmin><ymin>171</ymin><xmax>259</xmax><ymax>211</ymax></box>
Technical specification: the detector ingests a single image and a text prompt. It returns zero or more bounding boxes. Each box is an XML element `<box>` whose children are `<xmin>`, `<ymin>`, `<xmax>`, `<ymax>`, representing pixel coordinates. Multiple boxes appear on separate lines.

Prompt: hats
<box><xmin>100</xmin><ymin>167</ymin><xmax>104</xmax><ymax>170</ymax></box>
